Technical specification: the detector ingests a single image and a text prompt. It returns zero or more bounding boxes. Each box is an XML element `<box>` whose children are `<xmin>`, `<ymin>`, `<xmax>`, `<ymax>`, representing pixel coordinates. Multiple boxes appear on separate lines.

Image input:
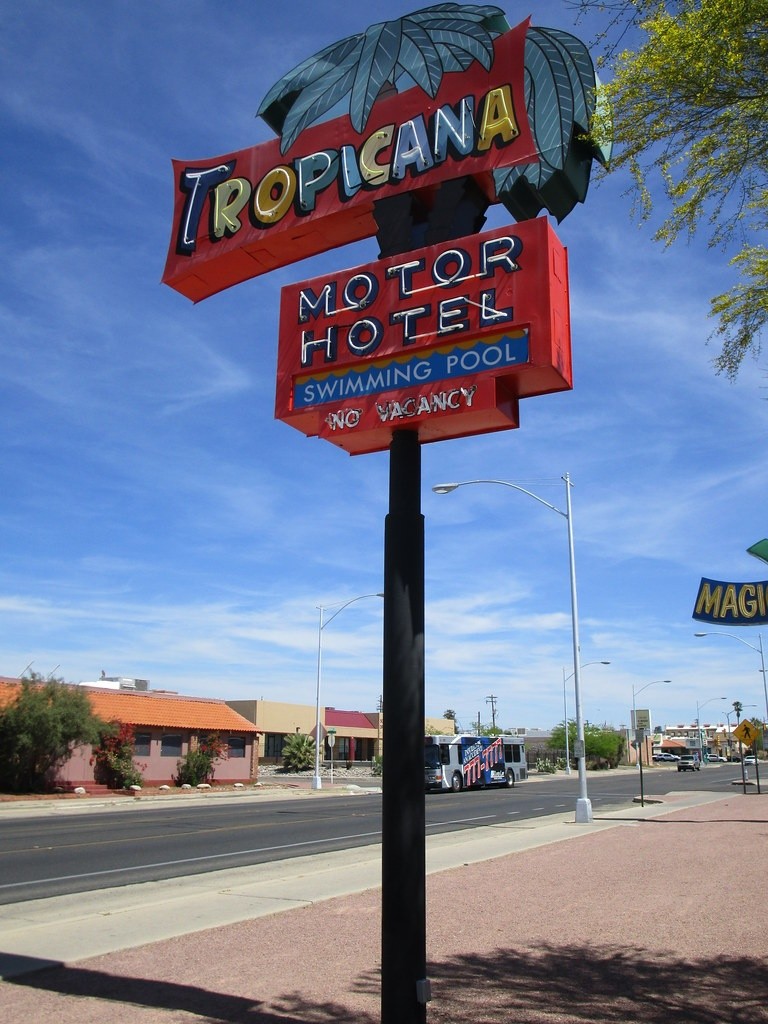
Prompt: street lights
<box><xmin>727</xmin><ymin>704</ymin><xmax>757</xmax><ymax>762</ymax></box>
<box><xmin>431</xmin><ymin>471</ymin><xmax>593</xmax><ymax>824</ymax></box>
<box><xmin>310</xmin><ymin>592</ymin><xmax>384</xmax><ymax>790</ymax></box>
<box><xmin>562</xmin><ymin>661</ymin><xmax>611</xmax><ymax>774</ymax></box>
<box><xmin>697</xmin><ymin>696</ymin><xmax>729</xmax><ymax>764</ymax></box>
<box><xmin>695</xmin><ymin>631</ymin><xmax>767</xmax><ymax>717</ymax></box>
<box><xmin>633</xmin><ymin>679</ymin><xmax>672</xmax><ymax>770</ymax></box>
<box><xmin>726</xmin><ymin>703</ymin><xmax>757</xmax><ymax>762</ymax></box>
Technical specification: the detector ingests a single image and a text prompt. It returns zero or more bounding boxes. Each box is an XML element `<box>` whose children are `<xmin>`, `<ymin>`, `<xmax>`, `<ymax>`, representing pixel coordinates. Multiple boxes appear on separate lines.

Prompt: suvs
<box><xmin>704</xmin><ymin>753</ymin><xmax>727</xmax><ymax>762</ymax></box>
<box><xmin>677</xmin><ymin>754</ymin><xmax>700</xmax><ymax>772</ymax></box>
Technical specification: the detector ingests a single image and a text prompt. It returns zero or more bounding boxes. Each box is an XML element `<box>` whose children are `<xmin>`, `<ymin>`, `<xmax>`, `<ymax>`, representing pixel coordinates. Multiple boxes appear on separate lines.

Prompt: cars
<box><xmin>744</xmin><ymin>755</ymin><xmax>760</xmax><ymax>766</ymax></box>
<box><xmin>727</xmin><ymin>755</ymin><xmax>742</xmax><ymax>763</ymax></box>
<box><xmin>652</xmin><ymin>752</ymin><xmax>681</xmax><ymax>762</ymax></box>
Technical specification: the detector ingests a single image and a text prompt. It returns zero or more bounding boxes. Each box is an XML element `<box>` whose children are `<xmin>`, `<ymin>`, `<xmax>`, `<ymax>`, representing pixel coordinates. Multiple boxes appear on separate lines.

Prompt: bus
<box><xmin>425</xmin><ymin>732</ymin><xmax>528</xmax><ymax>793</ymax></box>
